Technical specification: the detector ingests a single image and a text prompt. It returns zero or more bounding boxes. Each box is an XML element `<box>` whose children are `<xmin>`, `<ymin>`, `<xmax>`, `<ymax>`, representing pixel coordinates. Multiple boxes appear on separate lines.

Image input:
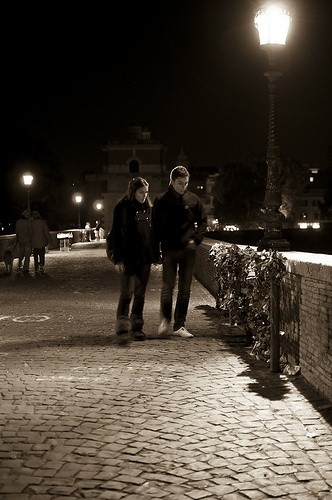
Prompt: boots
<box><xmin>130</xmin><ymin>316</ymin><xmax>145</xmax><ymax>340</ymax></box>
<box><xmin>116</xmin><ymin>315</ymin><xmax>131</xmax><ymax>343</ymax></box>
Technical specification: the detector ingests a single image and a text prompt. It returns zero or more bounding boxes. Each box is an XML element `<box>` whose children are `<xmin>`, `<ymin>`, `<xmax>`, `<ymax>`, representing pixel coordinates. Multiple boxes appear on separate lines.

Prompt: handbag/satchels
<box><xmin>106</xmin><ymin>234</ymin><xmax>116</xmax><ymax>261</ymax></box>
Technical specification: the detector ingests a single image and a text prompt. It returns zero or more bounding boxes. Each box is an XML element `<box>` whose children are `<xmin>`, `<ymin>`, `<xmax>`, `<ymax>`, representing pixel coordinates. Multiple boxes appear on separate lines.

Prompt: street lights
<box><xmin>96</xmin><ymin>203</ymin><xmax>102</xmax><ymax>231</ymax></box>
<box><xmin>23</xmin><ymin>172</ymin><xmax>34</xmax><ymax>209</ymax></box>
<box><xmin>254</xmin><ymin>2</ymin><xmax>293</xmax><ymax>252</ymax></box>
<box><xmin>75</xmin><ymin>196</ymin><xmax>81</xmax><ymax>228</ymax></box>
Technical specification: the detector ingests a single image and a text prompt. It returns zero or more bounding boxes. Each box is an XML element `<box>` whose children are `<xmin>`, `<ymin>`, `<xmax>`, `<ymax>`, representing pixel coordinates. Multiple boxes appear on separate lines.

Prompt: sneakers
<box><xmin>157</xmin><ymin>316</ymin><xmax>170</xmax><ymax>335</ymax></box>
<box><xmin>172</xmin><ymin>327</ymin><xmax>193</xmax><ymax>338</ymax></box>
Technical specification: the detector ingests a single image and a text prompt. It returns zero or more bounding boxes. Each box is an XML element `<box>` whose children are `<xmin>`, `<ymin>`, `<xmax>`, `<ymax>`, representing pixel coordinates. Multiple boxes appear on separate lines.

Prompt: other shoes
<box><xmin>23</xmin><ymin>270</ymin><xmax>28</xmax><ymax>273</ymax></box>
<box><xmin>39</xmin><ymin>264</ymin><xmax>45</xmax><ymax>273</ymax></box>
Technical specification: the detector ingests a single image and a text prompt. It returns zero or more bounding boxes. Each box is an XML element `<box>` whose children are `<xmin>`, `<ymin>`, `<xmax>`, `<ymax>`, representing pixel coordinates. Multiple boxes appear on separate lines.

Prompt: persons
<box><xmin>16</xmin><ymin>209</ymin><xmax>50</xmax><ymax>275</ymax></box>
<box><xmin>85</xmin><ymin>220</ymin><xmax>101</xmax><ymax>242</ymax></box>
<box><xmin>107</xmin><ymin>176</ymin><xmax>162</xmax><ymax>341</ymax></box>
<box><xmin>152</xmin><ymin>165</ymin><xmax>207</xmax><ymax>339</ymax></box>
